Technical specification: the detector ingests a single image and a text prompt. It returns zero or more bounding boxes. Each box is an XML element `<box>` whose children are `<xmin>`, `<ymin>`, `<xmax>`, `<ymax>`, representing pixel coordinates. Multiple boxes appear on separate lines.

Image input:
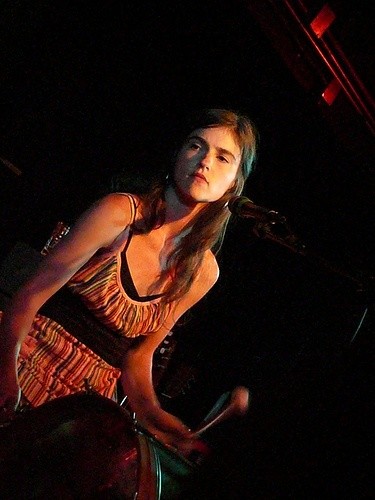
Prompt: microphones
<box><xmin>228</xmin><ymin>196</ymin><xmax>281</xmax><ymax>223</ymax></box>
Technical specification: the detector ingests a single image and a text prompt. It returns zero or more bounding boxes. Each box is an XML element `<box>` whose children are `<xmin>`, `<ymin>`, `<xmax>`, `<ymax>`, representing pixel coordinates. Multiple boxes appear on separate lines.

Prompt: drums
<box><xmin>0</xmin><ymin>391</ymin><xmax>209</xmax><ymax>500</ymax></box>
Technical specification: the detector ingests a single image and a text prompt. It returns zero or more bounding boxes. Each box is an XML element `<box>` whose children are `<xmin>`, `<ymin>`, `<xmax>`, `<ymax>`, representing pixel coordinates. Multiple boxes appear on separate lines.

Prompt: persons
<box><xmin>0</xmin><ymin>110</ymin><xmax>256</xmax><ymax>458</ymax></box>
